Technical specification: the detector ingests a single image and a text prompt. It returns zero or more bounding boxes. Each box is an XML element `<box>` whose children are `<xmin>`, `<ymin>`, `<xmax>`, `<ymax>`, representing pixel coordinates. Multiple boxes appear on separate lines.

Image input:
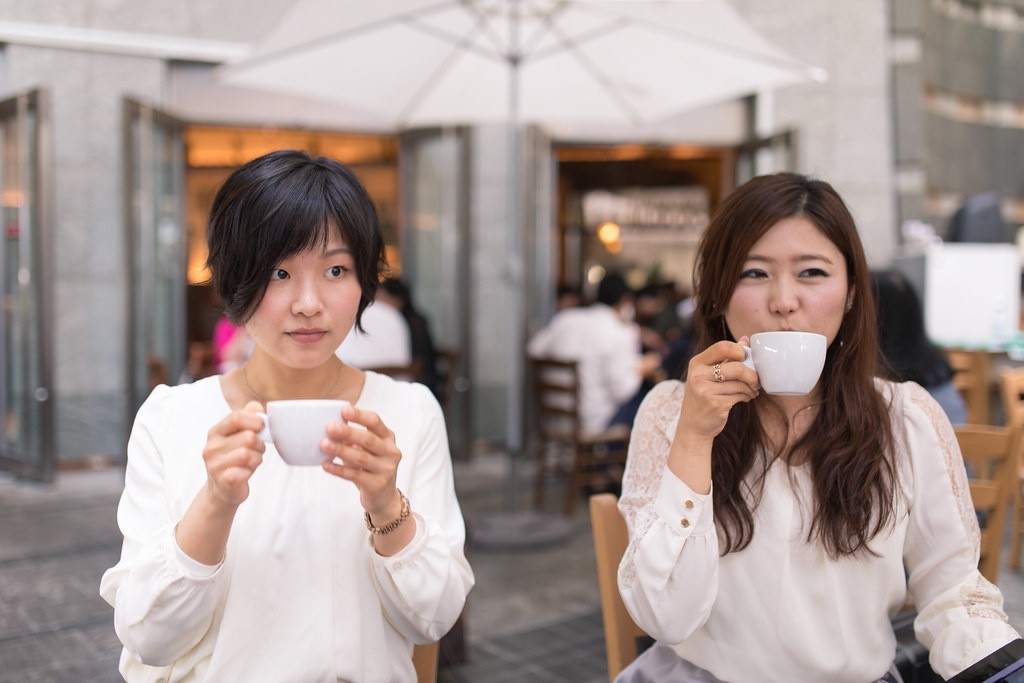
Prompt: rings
<box><xmin>713</xmin><ymin>365</ymin><xmax>722</xmax><ymax>381</ymax></box>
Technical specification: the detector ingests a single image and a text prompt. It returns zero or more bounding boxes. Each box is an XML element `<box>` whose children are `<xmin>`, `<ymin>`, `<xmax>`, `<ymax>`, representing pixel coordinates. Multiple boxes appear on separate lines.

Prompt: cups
<box><xmin>743</xmin><ymin>331</ymin><xmax>826</xmax><ymax>396</ymax></box>
<box><xmin>255</xmin><ymin>399</ymin><xmax>351</xmax><ymax>466</ymax></box>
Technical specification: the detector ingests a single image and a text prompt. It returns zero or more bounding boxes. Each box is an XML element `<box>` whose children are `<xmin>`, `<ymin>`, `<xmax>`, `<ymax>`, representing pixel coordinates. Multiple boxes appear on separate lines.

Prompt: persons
<box><xmin>609</xmin><ymin>173</ymin><xmax>1022</xmax><ymax>683</ymax></box>
<box><xmin>527</xmin><ymin>269</ymin><xmax>988</xmax><ymax>532</ymax></box>
<box><xmin>182</xmin><ymin>277</ymin><xmax>439</xmax><ymax>408</ymax></box>
<box><xmin>100</xmin><ymin>149</ymin><xmax>477</xmax><ymax>683</ymax></box>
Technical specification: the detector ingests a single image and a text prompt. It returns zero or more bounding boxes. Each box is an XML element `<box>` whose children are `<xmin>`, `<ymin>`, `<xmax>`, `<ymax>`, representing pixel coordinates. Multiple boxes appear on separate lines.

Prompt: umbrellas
<box><xmin>226</xmin><ymin>0</ymin><xmax>822</xmax><ymax>331</ymax></box>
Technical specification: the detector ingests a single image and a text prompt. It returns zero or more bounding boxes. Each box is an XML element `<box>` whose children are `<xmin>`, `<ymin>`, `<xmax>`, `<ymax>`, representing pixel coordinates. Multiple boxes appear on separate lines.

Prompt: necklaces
<box><xmin>244</xmin><ymin>364</ymin><xmax>342</xmax><ymax>403</ymax></box>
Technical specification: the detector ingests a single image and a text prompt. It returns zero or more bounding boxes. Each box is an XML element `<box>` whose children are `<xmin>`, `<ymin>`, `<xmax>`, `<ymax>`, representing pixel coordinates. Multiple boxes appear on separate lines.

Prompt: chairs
<box><xmin>997</xmin><ymin>367</ymin><xmax>1024</xmax><ymax>573</ymax></box>
<box><xmin>521</xmin><ymin>353</ymin><xmax>631</xmax><ymax>517</ymax></box>
<box><xmin>587</xmin><ymin>491</ymin><xmax>650</xmax><ymax>682</ymax></box>
<box><xmin>903</xmin><ymin>416</ymin><xmax>1024</xmax><ymax>612</ymax></box>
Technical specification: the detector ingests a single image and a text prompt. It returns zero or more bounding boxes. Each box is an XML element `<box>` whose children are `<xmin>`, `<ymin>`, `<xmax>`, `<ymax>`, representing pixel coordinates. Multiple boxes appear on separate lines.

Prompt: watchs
<box><xmin>364</xmin><ymin>490</ymin><xmax>410</xmax><ymax>534</ymax></box>
